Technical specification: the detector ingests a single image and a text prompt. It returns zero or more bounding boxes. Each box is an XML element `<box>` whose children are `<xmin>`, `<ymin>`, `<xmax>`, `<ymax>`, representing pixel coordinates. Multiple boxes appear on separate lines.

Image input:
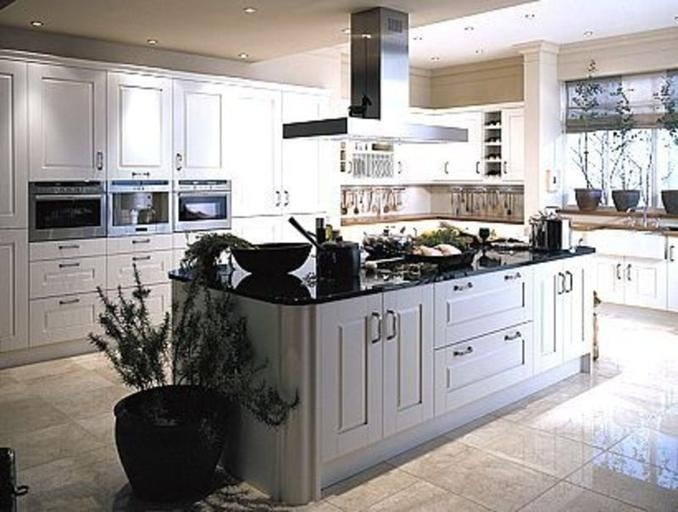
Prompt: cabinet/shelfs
<box><xmin>0</xmin><ymin>48</ymin><xmax>594</xmax><ymax>487</ymax></box>
<box><xmin>593</xmin><ymin>229</ymin><xmax>667</xmax><ymax>311</ymax></box>
<box><xmin>667</xmin><ymin>236</ymin><xmax>678</xmax><ymax>312</ymax></box>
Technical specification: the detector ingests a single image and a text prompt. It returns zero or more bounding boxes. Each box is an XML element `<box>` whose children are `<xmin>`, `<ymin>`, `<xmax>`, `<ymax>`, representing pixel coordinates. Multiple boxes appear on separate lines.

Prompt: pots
<box><xmin>288</xmin><ymin>216</ymin><xmax>363</xmax><ymax>280</ymax></box>
<box><xmin>529</xmin><ymin>216</ymin><xmax>564</xmax><ymax>252</ymax></box>
<box><xmin>364</xmin><ymin>223</ymin><xmax>414</xmax><ymax>257</ymax></box>
<box><xmin>225</xmin><ymin>232</ymin><xmax>313</xmax><ymax>279</ymax></box>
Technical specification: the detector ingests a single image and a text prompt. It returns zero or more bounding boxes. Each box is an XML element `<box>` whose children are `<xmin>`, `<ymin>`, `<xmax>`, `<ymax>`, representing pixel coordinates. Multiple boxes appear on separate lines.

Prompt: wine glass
<box><xmin>477</xmin><ymin>228</ymin><xmax>491</xmax><ymax>251</ymax></box>
<box><xmin>479</xmin><ymin>250</ymin><xmax>489</xmax><ymax>266</ymax></box>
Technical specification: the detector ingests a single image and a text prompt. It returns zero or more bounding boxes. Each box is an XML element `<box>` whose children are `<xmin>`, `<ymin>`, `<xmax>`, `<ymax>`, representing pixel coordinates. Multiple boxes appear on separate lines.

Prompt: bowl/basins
<box><xmin>406</xmin><ymin>247</ymin><xmax>476</xmax><ymax>272</ymax></box>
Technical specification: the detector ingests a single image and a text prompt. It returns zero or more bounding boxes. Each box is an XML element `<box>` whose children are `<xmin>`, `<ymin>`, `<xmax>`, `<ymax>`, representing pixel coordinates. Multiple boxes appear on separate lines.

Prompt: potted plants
<box><xmin>610</xmin><ymin>83</ymin><xmax>642</xmax><ymax>212</ymax></box>
<box><xmin>652</xmin><ymin>74</ymin><xmax>678</xmax><ymax>215</ymax></box>
<box><xmin>568</xmin><ymin>58</ymin><xmax>604</xmax><ymax>212</ymax></box>
<box><xmin>88</xmin><ymin>261</ymin><xmax>301</xmax><ymax>505</ymax></box>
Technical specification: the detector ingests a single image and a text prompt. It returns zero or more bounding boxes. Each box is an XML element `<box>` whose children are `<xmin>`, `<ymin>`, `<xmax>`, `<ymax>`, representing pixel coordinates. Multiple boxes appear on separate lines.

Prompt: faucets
<box><xmin>626</xmin><ymin>205</ymin><xmax>648</xmax><ymax>228</ymax></box>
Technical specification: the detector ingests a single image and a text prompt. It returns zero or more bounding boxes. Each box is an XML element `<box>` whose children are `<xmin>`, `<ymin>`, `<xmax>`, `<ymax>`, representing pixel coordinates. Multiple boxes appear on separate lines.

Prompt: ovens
<box><xmin>28</xmin><ymin>180</ymin><xmax>232</xmax><ymax>243</ymax></box>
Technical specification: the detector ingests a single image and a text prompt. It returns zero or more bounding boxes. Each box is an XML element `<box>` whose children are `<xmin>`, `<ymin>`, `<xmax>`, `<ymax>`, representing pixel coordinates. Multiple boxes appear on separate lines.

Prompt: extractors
<box><xmin>281</xmin><ymin>7</ymin><xmax>469</xmax><ymax>143</ymax></box>
